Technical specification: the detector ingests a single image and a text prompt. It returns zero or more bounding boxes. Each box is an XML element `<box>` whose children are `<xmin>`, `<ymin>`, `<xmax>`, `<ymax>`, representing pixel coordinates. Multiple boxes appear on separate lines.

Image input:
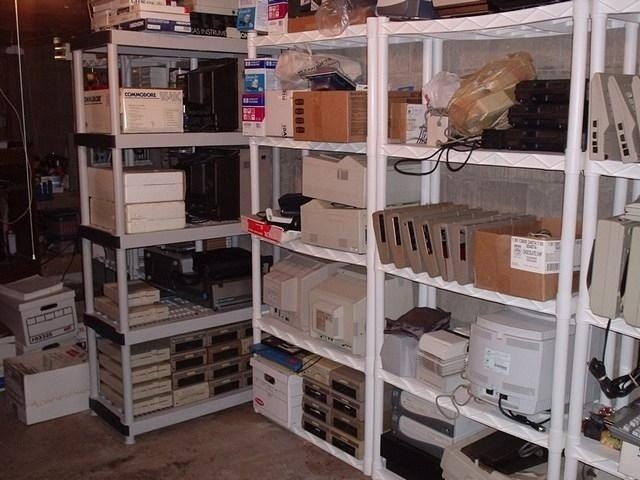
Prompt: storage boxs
<box><xmin>293</xmin><ymin>89</ymin><xmax>422</xmax><ymax>143</ymax></box>
<box><xmin>242</xmin><ymin>59</ymin><xmax>310</xmax><ymax>136</ymax></box>
<box><xmin>84</xmin><ymin>88</ymin><xmax>184</xmax><ymax>133</ymax></box>
<box><xmin>250</xmin><ymin>355</ymin><xmax>365</xmax><ymax>464</ymax></box>
<box><xmin>475</xmin><ymin>217</ymin><xmax>582</xmax><ymax>303</ymax></box>
<box><xmin>0</xmin><ymin>287</ymin><xmax>91</xmax><ymax>426</ymax></box>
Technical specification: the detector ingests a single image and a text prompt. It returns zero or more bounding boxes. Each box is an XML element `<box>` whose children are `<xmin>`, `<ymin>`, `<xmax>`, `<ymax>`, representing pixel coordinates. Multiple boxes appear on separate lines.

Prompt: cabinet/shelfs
<box><xmin>245</xmin><ymin>16</ymin><xmax>375</xmax><ymax>474</ymax></box>
<box><xmin>70</xmin><ymin>30</ymin><xmax>283</xmax><ymax>445</ymax></box>
<box><xmin>563</xmin><ymin>1</ymin><xmax>640</xmax><ymax>479</ymax></box>
<box><xmin>371</xmin><ymin>0</ymin><xmax>589</xmax><ymax>480</ymax></box>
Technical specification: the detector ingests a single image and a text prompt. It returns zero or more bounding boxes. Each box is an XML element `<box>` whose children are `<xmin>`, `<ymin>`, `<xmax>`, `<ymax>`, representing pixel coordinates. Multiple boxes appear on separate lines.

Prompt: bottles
<box><xmin>6</xmin><ymin>225</ymin><xmax>17</xmax><ymax>256</ymax></box>
<box><xmin>47</xmin><ymin>152</ymin><xmax>64</xmax><ymax>176</ymax></box>
